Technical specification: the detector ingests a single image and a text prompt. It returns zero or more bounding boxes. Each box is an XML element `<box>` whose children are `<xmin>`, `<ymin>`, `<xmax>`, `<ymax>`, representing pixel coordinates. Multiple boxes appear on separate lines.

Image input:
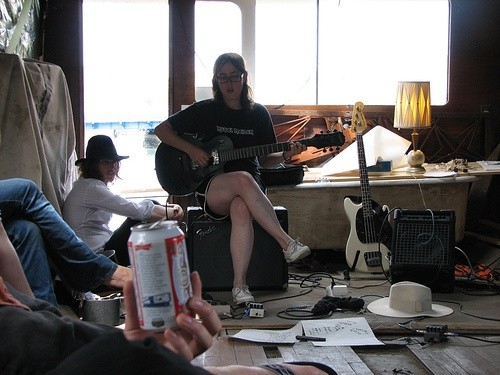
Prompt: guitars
<box><xmin>343</xmin><ymin>100</ymin><xmax>394</xmax><ymax>274</ymax></box>
<box><xmin>154</xmin><ymin>127</ymin><xmax>346</xmax><ymax>196</ymax></box>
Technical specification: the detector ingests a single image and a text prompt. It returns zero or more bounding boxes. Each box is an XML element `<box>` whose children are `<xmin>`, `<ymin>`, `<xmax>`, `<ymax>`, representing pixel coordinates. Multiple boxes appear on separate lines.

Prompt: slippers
<box><xmin>258</xmin><ymin>361</ymin><xmax>337</xmax><ymax>375</ymax></box>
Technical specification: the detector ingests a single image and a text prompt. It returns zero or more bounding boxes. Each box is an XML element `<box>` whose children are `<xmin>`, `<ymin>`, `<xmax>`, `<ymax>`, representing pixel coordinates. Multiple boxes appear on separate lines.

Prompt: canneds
<box><xmin>126</xmin><ymin>217</ymin><xmax>196</xmax><ymax>332</ymax></box>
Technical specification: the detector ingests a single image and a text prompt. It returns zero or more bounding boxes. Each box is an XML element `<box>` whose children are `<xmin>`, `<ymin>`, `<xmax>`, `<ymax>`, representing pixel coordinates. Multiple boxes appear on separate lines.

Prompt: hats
<box><xmin>75</xmin><ymin>135</ymin><xmax>129</xmax><ymax>166</ymax></box>
<box><xmin>366</xmin><ymin>280</ymin><xmax>454</xmax><ymax>318</ymax></box>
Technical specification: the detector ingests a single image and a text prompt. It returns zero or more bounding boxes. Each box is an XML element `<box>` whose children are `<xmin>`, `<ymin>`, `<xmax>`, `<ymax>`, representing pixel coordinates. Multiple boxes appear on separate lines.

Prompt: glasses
<box><xmin>215</xmin><ymin>72</ymin><xmax>243</xmax><ymax>83</ymax></box>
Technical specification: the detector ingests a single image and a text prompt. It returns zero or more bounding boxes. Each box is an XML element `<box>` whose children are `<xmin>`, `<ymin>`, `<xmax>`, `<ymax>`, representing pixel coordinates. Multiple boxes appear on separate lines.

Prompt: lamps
<box><xmin>393</xmin><ymin>80</ymin><xmax>432</xmax><ymax>167</ymax></box>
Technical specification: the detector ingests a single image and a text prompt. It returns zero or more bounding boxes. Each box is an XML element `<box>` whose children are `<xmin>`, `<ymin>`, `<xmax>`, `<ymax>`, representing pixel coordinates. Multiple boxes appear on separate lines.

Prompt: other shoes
<box><xmin>93</xmin><ymin>285</ymin><xmax>124</xmax><ymax>296</ymax></box>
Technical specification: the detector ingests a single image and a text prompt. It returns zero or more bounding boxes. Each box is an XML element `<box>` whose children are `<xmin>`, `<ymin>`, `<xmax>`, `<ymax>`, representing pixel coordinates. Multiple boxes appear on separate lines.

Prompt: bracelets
<box><xmin>283</xmin><ymin>152</ymin><xmax>291</xmax><ymax>161</ymax></box>
<box><xmin>165</xmin><ymin>206</ymin><xmax>169</xmax><ymax>219</ymax></box>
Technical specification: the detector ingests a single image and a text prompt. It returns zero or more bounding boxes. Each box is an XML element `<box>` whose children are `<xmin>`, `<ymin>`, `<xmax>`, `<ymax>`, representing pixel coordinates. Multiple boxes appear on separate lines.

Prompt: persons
<box><xmin>0</xmin><ymin>179</ymin><xmax>133</xmax><ymax>308</ymax></box>
<box><xmin>0</xmin><ymin>272</ymin><xmax>337</xmax><ymax>375</ymax></box>
<box><xmin>154</xmin><ymin>53</ymin><xmax>310</xmax><ymax>303</ymax></box>
<box><xmin>62</xmin><ymin>134</ymin><xmax>184</xmax><ymax>267</ymax></box>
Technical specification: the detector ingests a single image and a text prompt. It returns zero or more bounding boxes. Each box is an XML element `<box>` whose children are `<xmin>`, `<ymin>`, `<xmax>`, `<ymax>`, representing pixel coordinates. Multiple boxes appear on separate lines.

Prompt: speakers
<box><xmin>187</xmin><ymin>206</ymin><xmax>289</xmax><ymax>291</ymax></box>
<box><xmin>389</xmin><ymin>208</ymin><xmax>456</xmax><ymax>294</ymax></box>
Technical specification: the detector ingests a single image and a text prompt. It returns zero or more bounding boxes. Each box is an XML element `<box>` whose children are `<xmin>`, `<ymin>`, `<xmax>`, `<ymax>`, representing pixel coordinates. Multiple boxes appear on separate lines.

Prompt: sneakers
<box><xmin>232</xmin><ymin>285</ymin><xmax>254</xmax><ymax>305</ymax></box>
<box><xmin>282</xmin><ymin>238</ymin><xmax>311</xmax><ymax>263</ymax></box>
